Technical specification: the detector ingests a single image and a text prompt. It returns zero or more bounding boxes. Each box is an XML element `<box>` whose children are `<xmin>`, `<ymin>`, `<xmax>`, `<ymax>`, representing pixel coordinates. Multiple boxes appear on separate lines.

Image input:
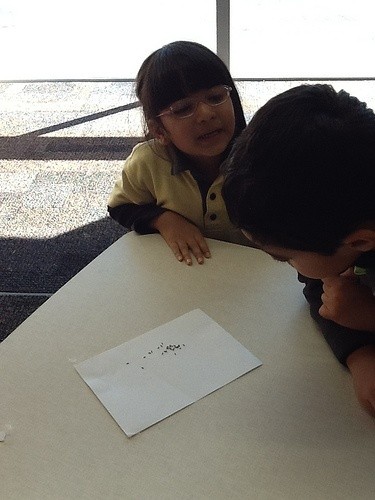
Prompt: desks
<box><xmin>1</xmin><ymin>228</ymin><xmax>375</xmax><ymax>500</ymax></box>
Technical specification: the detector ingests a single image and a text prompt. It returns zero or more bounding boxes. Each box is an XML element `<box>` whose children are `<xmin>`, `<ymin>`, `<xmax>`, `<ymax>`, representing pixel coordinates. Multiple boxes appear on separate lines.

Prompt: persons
<box><xmin>105</xmin><ymin>40</ymin><xmax>248</xmax><ymax>266</ymax></box>
<box><xmin>219</xmin><ymin>79</ymin><xmax>375</xmax><ymax>420</ymax></box>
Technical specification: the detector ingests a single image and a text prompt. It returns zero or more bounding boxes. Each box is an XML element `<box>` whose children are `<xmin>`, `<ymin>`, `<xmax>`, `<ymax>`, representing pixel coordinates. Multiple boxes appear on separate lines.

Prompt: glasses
<box><xmin>154</xmin><ymin>85</ymin><xmax>234</xmax><ymax>119</ymax></box>
